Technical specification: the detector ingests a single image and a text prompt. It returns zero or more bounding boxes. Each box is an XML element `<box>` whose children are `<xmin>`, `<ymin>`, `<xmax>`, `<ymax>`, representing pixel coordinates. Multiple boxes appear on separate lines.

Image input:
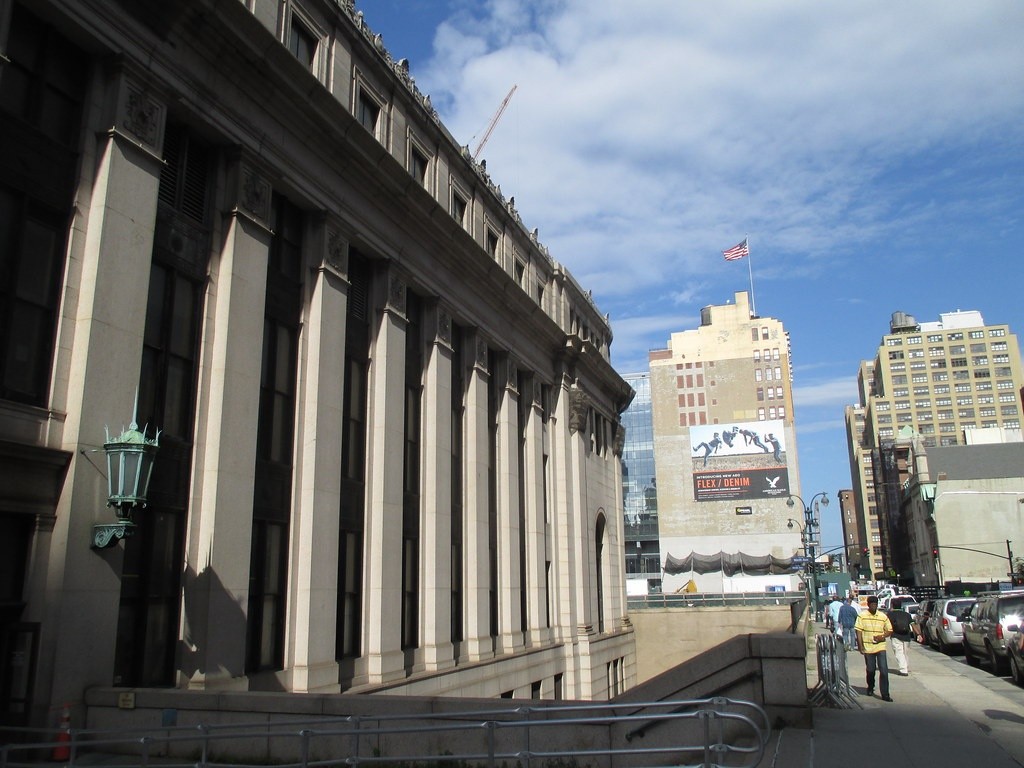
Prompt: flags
<box><xmin>722</xmin><ymin>237</ymin><xmax>748</xmax><ymax>262</ymax></box>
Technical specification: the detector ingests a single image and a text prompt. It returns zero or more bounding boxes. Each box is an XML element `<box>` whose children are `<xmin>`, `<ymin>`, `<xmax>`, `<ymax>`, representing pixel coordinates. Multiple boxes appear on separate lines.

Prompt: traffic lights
<box><xmin>933</xmin><ymin>546</ymin><xmax>939</xmax><ymax>558</ymax></box>
<box><xmin>1018</xmin><ymin>578</ymin><xmax>1023</xmax><ymax>585</ymax></box>
<box><xmin>863</xmin><ymin>547</ymin><xmax>868</xmax><ymax>558</ymax></box>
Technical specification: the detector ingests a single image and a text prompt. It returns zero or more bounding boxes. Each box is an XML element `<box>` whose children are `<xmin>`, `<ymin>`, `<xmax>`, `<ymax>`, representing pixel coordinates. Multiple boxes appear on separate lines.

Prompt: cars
<box><xmin>877</xmin><ymin>590</ymin><xmax>1024</xmax><ymax>686</ymax></box>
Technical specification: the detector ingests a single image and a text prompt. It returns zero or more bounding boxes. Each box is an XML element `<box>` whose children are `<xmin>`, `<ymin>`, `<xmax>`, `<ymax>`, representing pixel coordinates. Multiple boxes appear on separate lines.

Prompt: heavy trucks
<box><xmin>878</xmin><ymin>583</ymin><xmax>948</xmax><ymax>599</ymax></box>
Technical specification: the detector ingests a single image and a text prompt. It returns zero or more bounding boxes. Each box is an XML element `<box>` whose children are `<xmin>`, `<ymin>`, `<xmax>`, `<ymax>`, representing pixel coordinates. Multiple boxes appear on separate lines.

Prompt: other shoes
<box><xmin>867</xmin><ymin>688</ymin><xmax>873</xmax><ymax>695</ymax></box>
<box><xmin>882</xmin><ymin>697</ymin><xmax>893</xmax><ymax>702</ymax></box>
<box><xmin>898</xmin><ymin>673</ymin><xmax>908</xmax><ymax>676</ymax></box>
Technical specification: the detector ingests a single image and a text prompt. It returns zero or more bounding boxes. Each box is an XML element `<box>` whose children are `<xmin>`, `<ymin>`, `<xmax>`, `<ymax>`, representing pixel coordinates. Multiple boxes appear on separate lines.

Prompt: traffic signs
<box><xmin>790</xmin><ymin>557</ymin><xmax>813</xmax><ymax>562</ymax></box>
<box><xmin>787</xmin><ymin>566</ymin><xmax>807</xmax><ymax>570</ymax></box>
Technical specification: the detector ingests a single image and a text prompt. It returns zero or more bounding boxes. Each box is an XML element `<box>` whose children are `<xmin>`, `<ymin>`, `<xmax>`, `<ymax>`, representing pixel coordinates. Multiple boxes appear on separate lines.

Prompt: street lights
<box><xmin>788</xmin><ymin>517</ymin><xmax>820</xmax><ymax>618</ymax></box>
<box><xmin>786</xmin><ymin>492</ymin><xmax>830</xmax><ymax>622</ymax></box>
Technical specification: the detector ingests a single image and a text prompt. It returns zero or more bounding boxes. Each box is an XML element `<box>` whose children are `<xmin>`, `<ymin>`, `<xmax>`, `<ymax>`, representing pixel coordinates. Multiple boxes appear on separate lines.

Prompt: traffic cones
<box><xmin>52</xmin><ymin>701</ymin><xmax>74</xmax><ymax>761</ymax></box>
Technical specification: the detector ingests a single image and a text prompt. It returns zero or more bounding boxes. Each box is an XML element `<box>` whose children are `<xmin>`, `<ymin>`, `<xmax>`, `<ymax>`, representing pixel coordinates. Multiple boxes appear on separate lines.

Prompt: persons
<box><xmin>692</xmin><ymin>432</ymin><xmax>722</xmax><ymax>469</ymax></box>
<box><xmin>764</xmin><ymin>433</ymin><xmax>783</xmax><ymax>466</ymax></box>
<box><xmin>722</xmin><ymin>426</ymin><xmax>739</xmax><ymax>448</ymax></box>
<box><xmin>739</xmin><ymin>428</ymin><xmax>768</xmax><ymax>454</ymax></box>
<box><xmin>887</xmin><ymin>598</ymin><xmax>924</xmax><ymax>676</ymax></box>
<box><xmin>853</xmin><ymin>595</ymin><xmax>894</xmax><ymax>702</ymax></box>
<box><xmin>824</xmin><ymin>594</ymin><xmax>857</xmax><ymax>652</ymax></box>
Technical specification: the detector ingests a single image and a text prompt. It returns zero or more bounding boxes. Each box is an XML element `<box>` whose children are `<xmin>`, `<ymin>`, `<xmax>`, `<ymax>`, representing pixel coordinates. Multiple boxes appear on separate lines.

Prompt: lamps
<box><xmin>88</xmin><ymin>384</ymin><xmax>164</xmax><ymax>550</ymax></box>
